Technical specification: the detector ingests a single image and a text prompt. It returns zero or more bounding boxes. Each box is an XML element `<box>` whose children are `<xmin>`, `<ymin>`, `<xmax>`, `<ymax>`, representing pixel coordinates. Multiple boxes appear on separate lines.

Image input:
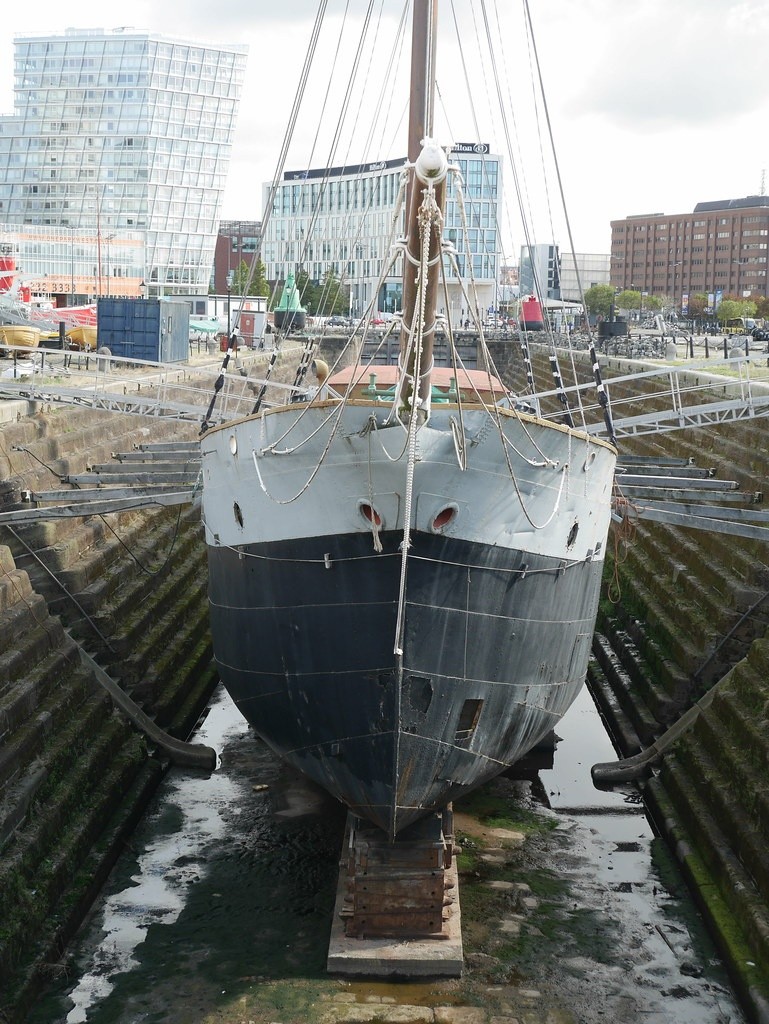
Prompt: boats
<box><xmin>186</xmin><ymin>316</ymin><xmax>221</xmax><ymax>341</ymax></box>
<box><xmin>0</xmin><ymin>324</ymin><xmax>39</xmax><ymax>357</ymax></box>
<box><xmin>65</xmin><ymin>326</ymin><xmax>97</xmax><ymax>351</ymax></box>
<box><xmin>274</xmin><ymin>267</ymin><xmax>307</xmax><ymax>330</ymax></box>
<box><xmin>184</xmin><ymin>0</ymin><xmax>611</xmax><ymax>840</ymax></box>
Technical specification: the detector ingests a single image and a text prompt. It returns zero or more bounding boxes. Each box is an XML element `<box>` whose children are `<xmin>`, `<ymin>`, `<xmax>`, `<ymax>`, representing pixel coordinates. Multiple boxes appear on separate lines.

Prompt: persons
<box><xmin>460</xmin><ymin>319</ymin><xmax>462</xmax><ymax>326</ymax></box>
<box><xmin>464</xmin><ymin>318</ymin><xmax>470</xmax><ymax>330</ymax></box>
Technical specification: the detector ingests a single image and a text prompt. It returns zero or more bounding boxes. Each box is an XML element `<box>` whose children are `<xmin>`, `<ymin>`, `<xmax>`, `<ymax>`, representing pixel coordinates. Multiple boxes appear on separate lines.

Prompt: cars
<box><xmin>323</xmin><ymin>316</ymin><xmax>342</xmax><ymax>326</ymax></box>
<box><xmin>328</xmin><ymin>316</ymin><xmax>349</xmax><ymax>326</ymax></box>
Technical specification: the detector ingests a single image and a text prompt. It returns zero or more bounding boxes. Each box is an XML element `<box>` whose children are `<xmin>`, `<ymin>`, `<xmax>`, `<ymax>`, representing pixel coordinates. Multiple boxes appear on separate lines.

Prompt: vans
<box><xmin>488</xmin><ymin>319</ymin><xmax>505</xmax><ymax>328</ymax></box>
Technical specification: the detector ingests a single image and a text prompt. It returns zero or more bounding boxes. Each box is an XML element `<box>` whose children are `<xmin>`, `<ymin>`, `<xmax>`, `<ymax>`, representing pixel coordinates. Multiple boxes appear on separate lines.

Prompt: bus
<box><xmin>722</xmin><ymin>317</ymin><xmax>755</xmax><ymax>335</ymax></box>
